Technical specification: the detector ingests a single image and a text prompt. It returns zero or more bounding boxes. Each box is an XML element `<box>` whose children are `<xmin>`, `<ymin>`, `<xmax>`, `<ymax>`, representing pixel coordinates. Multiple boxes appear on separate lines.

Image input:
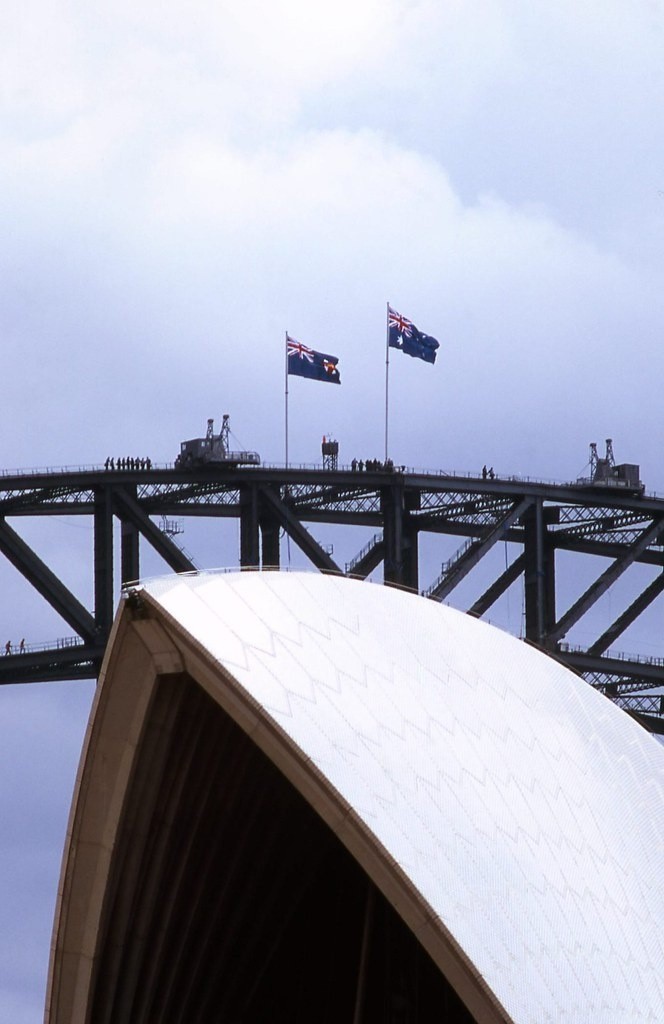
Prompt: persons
<box><xmin>105</xmin><ymin>456</ymin><xmax>151</xmax><ymax>472</ymax></box>
<box><xmin>5</xmin><ymin>640</ymin><xmax>11</xmax><ymax>656</ymax></box>
<box><xmin>351</xmin><ymin>458</ymin><xmax>393</xmax><ymax>472</ymax></box>
<box><xmin>20</xmin><ymin>638</ymin><xmax>25</xmax><ymax>654</ymax></box>
<box><xmin>482</xmin><ymin>465</ymin><xmax>495</xmax><ymax>480</ymax></box>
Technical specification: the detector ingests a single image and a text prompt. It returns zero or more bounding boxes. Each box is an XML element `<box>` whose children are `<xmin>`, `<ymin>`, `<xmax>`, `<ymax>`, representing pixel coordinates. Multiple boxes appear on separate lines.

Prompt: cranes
<box><xmin>589</xmin><ymin>443</ymin><xmax>599</xmax><ymax>485</ymax></box>
<box><xmin>605</xmin><ymin>439</ymin><xmax>616</xmax><ymax>468</ymax></box>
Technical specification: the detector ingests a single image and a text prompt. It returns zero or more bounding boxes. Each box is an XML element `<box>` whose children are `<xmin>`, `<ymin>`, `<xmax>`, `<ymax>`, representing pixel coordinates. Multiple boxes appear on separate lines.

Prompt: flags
<box><xmin>388</xmin><ymin>307</ymin><xmax>440</xmax><ymax>365</ymax></box>
<box><xmin>286</xmin><ymin>334</ymin><xmax>341</xmax><ymax>385</ymax></box>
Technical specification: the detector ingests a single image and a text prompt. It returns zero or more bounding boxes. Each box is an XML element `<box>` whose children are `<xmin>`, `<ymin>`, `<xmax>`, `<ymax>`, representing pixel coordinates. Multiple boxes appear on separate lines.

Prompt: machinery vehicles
<box><xmin>173</xmin><ymin>415</ymin><xmax>261</xmax><ymax>468</ymax></box>
